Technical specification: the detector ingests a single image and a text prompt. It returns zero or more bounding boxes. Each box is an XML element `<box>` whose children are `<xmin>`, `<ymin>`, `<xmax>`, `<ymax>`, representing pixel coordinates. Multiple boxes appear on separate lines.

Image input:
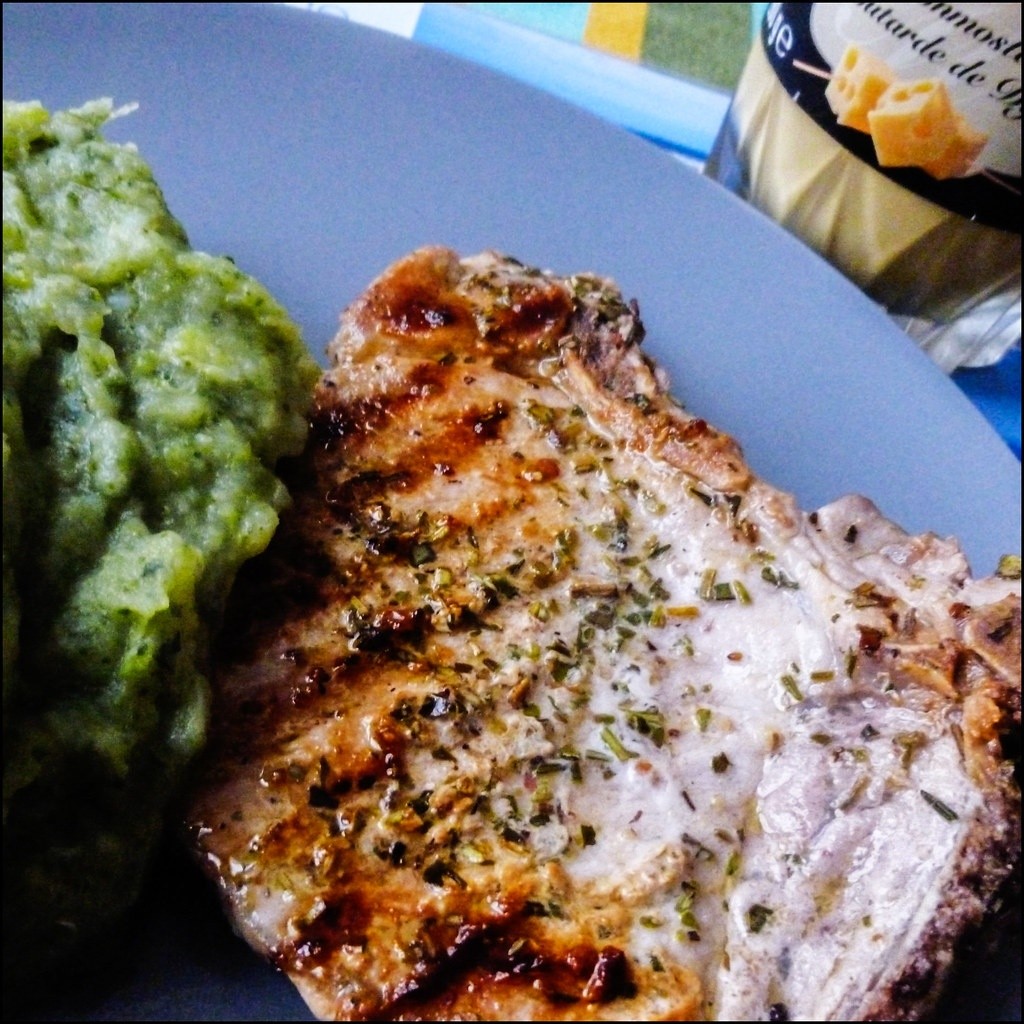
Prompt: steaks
<box><xmin>178</xmin><ymin>243</ymin><xmax>1024</xmax><ymax>1022</ymax></box>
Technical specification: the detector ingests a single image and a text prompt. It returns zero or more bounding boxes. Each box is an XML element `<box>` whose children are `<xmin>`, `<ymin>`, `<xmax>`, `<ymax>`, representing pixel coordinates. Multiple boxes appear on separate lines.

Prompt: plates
<box><xmin>0</xmin><ymin>0</ymin><xmax>1024</xmax><ymax>1024</ymax></box>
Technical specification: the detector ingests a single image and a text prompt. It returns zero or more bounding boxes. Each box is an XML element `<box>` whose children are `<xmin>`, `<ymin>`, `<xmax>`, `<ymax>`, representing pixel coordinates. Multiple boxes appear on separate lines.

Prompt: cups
<box><xmin>703</xmin><ymin>0</ymin><xmax>1024</xmax><ymax>378</ymax></box>
